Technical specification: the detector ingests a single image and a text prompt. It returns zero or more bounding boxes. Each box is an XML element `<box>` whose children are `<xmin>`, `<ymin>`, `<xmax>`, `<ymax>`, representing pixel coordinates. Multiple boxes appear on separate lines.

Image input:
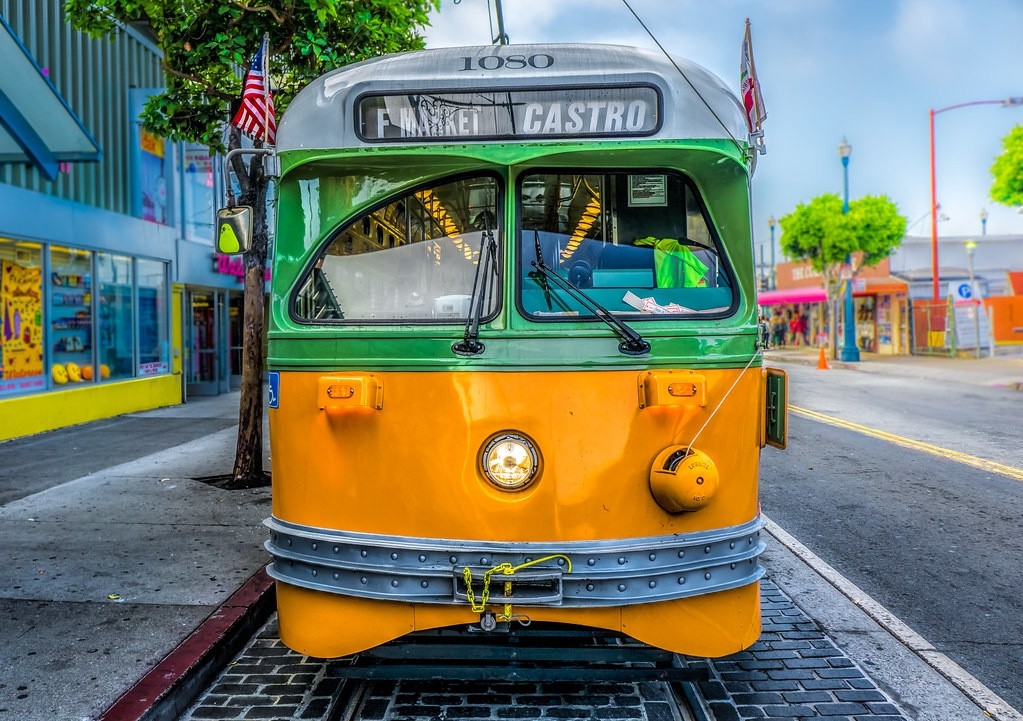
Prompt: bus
<box><xmin>210</xmin><ymin>0</ymin><xmax>793</xmax><ymax>690</ymax></box>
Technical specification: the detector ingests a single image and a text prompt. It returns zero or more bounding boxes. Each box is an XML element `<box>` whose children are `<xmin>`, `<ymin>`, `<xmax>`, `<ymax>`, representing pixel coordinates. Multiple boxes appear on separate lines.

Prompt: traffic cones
<box><xmin>814</xmin><ymin>345</ymin><xmax>833</xmax><ymax>371</ymax></box>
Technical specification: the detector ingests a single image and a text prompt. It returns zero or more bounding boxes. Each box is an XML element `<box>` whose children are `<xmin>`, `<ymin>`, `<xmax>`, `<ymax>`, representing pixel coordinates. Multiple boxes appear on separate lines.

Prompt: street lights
<box><xmin>836</xmin><ymin>136</ymin><xmax>863</xmax><ymax>364</ymax></box>
<box><xmin>978</xmin><ymin>206</ymin><xmax>988</xmax><ymax>234</ymax></box>
<box><xmin>928</xmin><ymin>97</ymin><xmax>1022</xmax><ymax>298</ymax></box>
<box><xmin>766</xmin><ymin>214</ymin><xmax>777</xmax><ymax>291</ymax></box>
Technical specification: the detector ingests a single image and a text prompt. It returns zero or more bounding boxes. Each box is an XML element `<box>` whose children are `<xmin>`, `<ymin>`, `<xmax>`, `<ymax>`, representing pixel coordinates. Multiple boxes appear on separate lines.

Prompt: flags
<box><xmin>738</xmin><ymin>28</ymin><xmax>767</xmax><ymax>132</ymax></box>
<box><xmin>232</xmin><ymin>36</ymin><xmax>276</xmax><ymax>143</ymax></box>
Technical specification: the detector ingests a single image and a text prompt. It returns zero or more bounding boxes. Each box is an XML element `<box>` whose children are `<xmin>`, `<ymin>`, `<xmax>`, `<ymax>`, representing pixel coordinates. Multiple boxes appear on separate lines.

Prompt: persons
<box><xmin>760</xmin><ymin>309</ymin><xmax>806</xmax><ymax>347</ymax></box>
